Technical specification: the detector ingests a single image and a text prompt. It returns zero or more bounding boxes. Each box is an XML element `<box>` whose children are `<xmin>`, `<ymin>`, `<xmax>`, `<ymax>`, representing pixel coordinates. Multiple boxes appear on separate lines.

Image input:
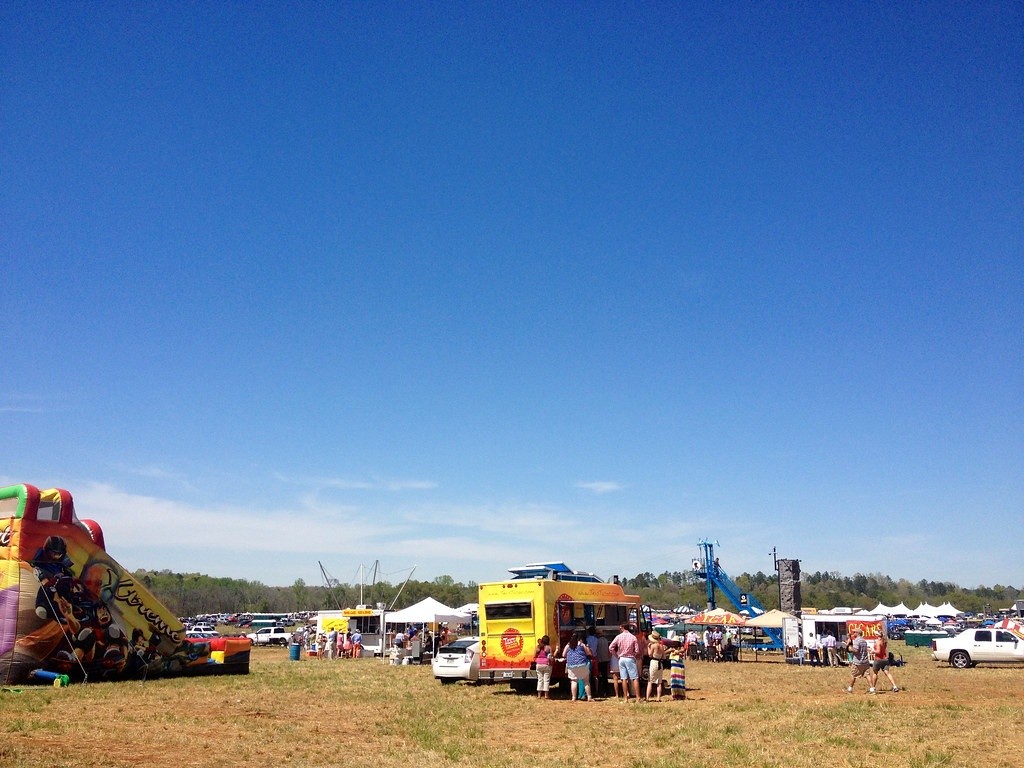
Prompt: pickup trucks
<box><xmin>276</xmin><ymin>617</ymin><xmax>295</xmax><ymax>627</ymax></box>
<box><xmin>933</xmin><ymin>627</ymin><xmax>1024</xmax><ymax>669</ymax></box>
<box><xmin>246</xmin><ymin>627</ymin><xmax>293</xmax><ymax>646</ymax></box>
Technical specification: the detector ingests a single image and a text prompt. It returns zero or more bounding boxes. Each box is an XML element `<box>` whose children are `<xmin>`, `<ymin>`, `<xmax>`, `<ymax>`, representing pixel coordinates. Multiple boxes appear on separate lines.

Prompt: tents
<box><xmin>683</xmin><ymin>607</ymin><xmax>751</xmax><ymax>663</ymax></box>
<box><xmin>867</xmin><ymin>598</ymin><xmax>963</xmax><ymax>617</ymax></box>
<box><xmin>744</xmin><ymin>609</ymin><xmax>802</xmax><ymax>663</ymax></box>
<box><xmin>381</xmin><ymin>595</ymin><xmax>475</xmax><ymax>666</ymax></box>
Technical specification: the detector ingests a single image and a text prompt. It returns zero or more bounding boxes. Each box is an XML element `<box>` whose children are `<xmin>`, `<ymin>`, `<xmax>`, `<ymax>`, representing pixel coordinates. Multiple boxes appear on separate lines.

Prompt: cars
<box><xmin>432</xmin><ymin>635</ymin><xmax>481</xmax><ymax>683</ymax></box>
<box><xmin>179</xmin><ymin>609</ymin><xmax>243</xmax><ymax>639</ymax></box>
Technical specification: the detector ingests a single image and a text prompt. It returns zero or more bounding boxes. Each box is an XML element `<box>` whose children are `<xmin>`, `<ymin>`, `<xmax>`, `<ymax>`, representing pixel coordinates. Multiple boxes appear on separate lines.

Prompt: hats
<box><xmin>873</xmin><ymin>631</ymin><xmax>883</xmax><ymax>636</ymax></box>
<box><xmin>852</xmin><ymin>628</ymin><xmax>863</xmax><ymax>634</ymax></box>
<box><xmin>648</xmin><ymin>630</ymin><xmax>660</xmax><ymax>643</ymax></box>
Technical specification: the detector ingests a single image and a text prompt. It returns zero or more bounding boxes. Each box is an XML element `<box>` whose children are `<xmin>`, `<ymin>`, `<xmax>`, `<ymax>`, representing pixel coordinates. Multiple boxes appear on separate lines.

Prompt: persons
<box><xmin>561</xmin><ymin>621</ymin><xmax>620</xmax><ymax>703</ymax></box>
<box><xmin>608</xmin><ymin>621</ymin><xmax>643</xmax><ymax>705</ymax></box>
<box><xmin>664</xmin><ymin>636</ymin><xmax>687</xmax><ymax>701</ymax></box>
<box><xmin>642</xmin><ymin>631</ymin><xmax>667</xmax><ymax>702</ymax></box>
<box><xmin>683</xmin><ymin>626</ymin><xmax>740</xmax><ymax>662</ymax></box>
<box><xmin>807</xmin><ymin>630</ymin><xmax>854</xmax><ymax>669</ymax></box>
<box><xmin>532</xmin><ymin>634</ymin><xmax>560</xmax><ymax>701</ymax></box>
<box><xmin>842</xmin><ymin>627</ymin><xmax>877</xmax><ymax>696</ymax></box>
<box><xmin>297</xmin><ymin>621</ymin><xmax>453</xmax><ymax>660</ymax></box>
<box><xmin>868</xmin><ymin>628</ymin><xmax>899</xmax><ymax>694</ymax></box>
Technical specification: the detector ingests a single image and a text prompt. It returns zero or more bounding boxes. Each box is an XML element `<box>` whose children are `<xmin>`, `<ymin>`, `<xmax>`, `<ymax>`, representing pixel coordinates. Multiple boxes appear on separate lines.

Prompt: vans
<box><xmin>475</xmin><ymin>562</ymin><xmax>654</xmax><ymax>693</ymax></box>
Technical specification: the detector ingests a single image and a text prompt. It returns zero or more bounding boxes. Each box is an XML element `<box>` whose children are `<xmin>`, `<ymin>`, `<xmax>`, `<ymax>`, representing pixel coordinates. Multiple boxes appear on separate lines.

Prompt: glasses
<box><xmin>853</xmin><ymin>631</ymin><xmax>858</xmax><ymax>633</ymax></box>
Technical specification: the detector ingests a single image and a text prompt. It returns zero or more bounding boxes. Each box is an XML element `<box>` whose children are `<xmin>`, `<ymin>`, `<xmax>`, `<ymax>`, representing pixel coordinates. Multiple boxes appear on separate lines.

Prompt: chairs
<box><xmin>785</xmin><ymin>645</ymin><xmax>876</xmax><ymax>662</ymax></box>
<box><xmin>688</xmin><ymin>643</ymin><xmax>740</xmax><ymax>663</ymax></box>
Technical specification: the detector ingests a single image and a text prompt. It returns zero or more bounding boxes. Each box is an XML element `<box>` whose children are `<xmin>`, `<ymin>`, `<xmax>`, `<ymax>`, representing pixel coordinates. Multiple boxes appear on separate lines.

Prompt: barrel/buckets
<box><xmin>290</xmin><ymin>644</ymin><xmax>299</xmax><ymax>661</ymax></box>
